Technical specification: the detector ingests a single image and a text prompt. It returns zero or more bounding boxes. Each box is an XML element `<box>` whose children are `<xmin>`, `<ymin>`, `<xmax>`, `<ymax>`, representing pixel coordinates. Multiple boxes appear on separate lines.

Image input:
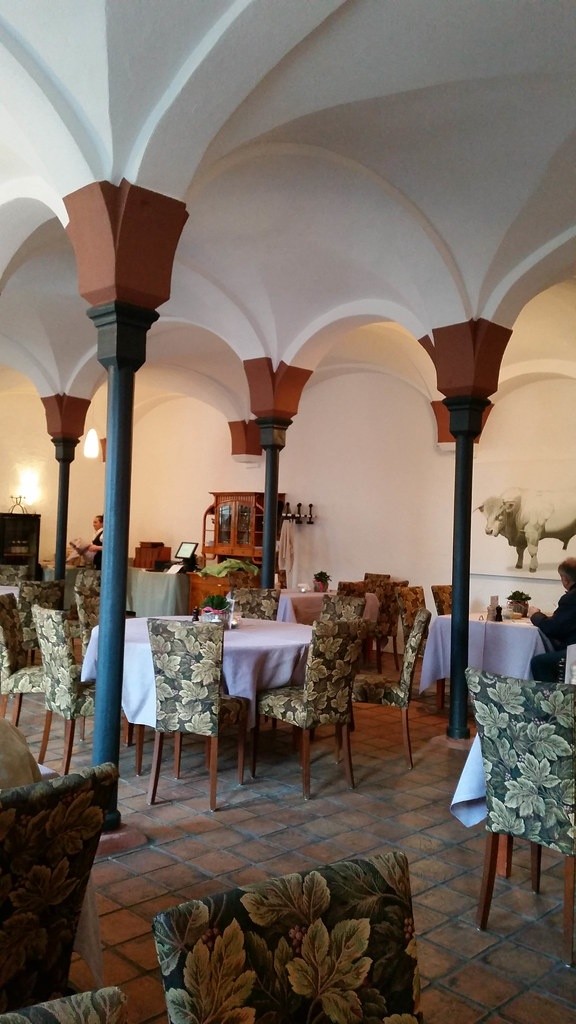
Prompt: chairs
<box><xmin>0</xmin><ymin>760</ymin><xmax>118</xmax><ymax>1013</ymax></box>
<box><xmin>337</xmin><ymin>572</ymin><xmax>409</xmax><ymax>676</ymax></box>
<box><xmin>430</xmin><ymin>585</ymin><xmax>452</xmax><ymax>615</ymax></box>
<box><xmin>16</xmin><ymin>577</ymin><xmax>66</xmax><ymax>651</ymax></box>
<box><xmin>30</xmin><ymin>601</ymin><xmax>146</xmax><ymax>777</ymax></box>
<box><xmin>0</xmin><ymin>592</ymin><xmax>85</xmax><ymax>742</ymax></box>
<box><xmin>0</xmin><ymin>563</ymin><xmax>30</xmax><ymax>588</ymax></box>
<box><xmin>334</xmin><ymin>608</ymin><xmax>434</xmax><ymax>768</ymax></box>
<box><xmin>394</xmin><ymin>585</ymin><xmax>446</xmax><ymax>709</ymax></box>
<box><xmin>249</xmin><ymin>618</ymin><xmax>370</xmax><ymax>805</ymax></box>
<box><xmin>229</xmin><ymin>586</ymin><xmax>282</xmax><ymax>621</ymax></box>
<box><xmin>0</xmin><ymin>985</ymin><xmax>129</xmax><ymax>1024</ymax></box>
<box><xmin>146</xmin><ymin>618</ymin><xmax>252</xmax><ymax>811</ymax></box>
<box><xmin>464</xmin><ymin>664</ymin><xmax>576</xmax><ymax>964</ymax></box>
<box><xmin>66</xmin><ymin>567</ymin><xmax>101</xmax><ymax>663</ymax></box>
<box><xmin>318</xmin><ymin>592</ymin><xmax>368</xmax><ymax>618</ymax></box>
<box><xmin>150</xmin><ymin>850</ymin><xmax>425</xmax><ymax>1024</ymax></box>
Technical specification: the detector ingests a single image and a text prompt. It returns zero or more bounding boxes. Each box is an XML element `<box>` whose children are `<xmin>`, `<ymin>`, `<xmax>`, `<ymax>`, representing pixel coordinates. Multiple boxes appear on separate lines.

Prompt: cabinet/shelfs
<box><xmin>201</xmin><ymin>489</ymin><xmax>286</xmax><ymax>564</ymax></box>
<box><xmin>186</xmin><ymin>570</ymin><xmax>261</xmax><ymax>614</ymax></box>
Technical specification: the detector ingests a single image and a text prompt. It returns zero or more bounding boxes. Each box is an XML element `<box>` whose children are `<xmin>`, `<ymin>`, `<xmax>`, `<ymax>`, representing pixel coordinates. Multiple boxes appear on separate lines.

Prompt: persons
<box><xmin>528</xmin><ymin>556</ymin><xmax>576</xmax><ymax>683</ymax></box>
<box><xmin>88</xmin><ymin>515</ymin><xmax>104</xmax><ymax>570</ymax></box>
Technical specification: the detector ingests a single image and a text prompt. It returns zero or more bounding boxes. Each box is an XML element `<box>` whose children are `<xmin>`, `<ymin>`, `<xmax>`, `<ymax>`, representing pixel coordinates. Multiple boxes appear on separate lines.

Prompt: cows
<box><xmin>472</xmin><ymin>486</ymin><xmax>576</xmax><ymax>573</ymax></box>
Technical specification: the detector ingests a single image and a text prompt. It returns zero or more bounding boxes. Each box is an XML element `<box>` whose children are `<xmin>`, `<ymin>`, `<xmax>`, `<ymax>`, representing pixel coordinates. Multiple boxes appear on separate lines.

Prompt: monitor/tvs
<box><xmin>175</xmin><ymin>542</ymin><xmax>199</xmax><ymax>559</ymax></box>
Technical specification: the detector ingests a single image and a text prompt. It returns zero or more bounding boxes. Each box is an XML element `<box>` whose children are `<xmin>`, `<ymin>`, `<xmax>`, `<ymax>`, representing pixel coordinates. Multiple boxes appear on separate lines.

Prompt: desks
<box><xmin>418</xmin><ymin>610</ymin><xmax>555</xmax><ymax>696</ymax></box>
<box><xmin>0</xmin><ymin>585</ymin><xmax>22</xmax><ymax>598</ymax></box>
<box><xmin>280</xmin><ymin>586</ymin><xmax>380</xmax><ymax>625</ymax></box>
<box><xmin>127</xmin><ymin>565</ymin><xmax>190</xmax><ymax>616</ymax></box>
<box><xmin>80</xmin><ymin>611</ymin><xmax>318</xmax><ymax>785</ymax></box>
<box><xmin>42</xmin><ymin>561</ymin><xmax>79</xmax><ymax>610</ymax></box>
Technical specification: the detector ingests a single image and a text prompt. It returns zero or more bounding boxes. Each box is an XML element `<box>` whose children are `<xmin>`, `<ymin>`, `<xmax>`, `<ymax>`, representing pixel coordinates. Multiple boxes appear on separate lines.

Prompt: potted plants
<box><xmin>313</xmin><ymin>571</ymin><xmax>332</xmax><ymax>593</ymax></box>
